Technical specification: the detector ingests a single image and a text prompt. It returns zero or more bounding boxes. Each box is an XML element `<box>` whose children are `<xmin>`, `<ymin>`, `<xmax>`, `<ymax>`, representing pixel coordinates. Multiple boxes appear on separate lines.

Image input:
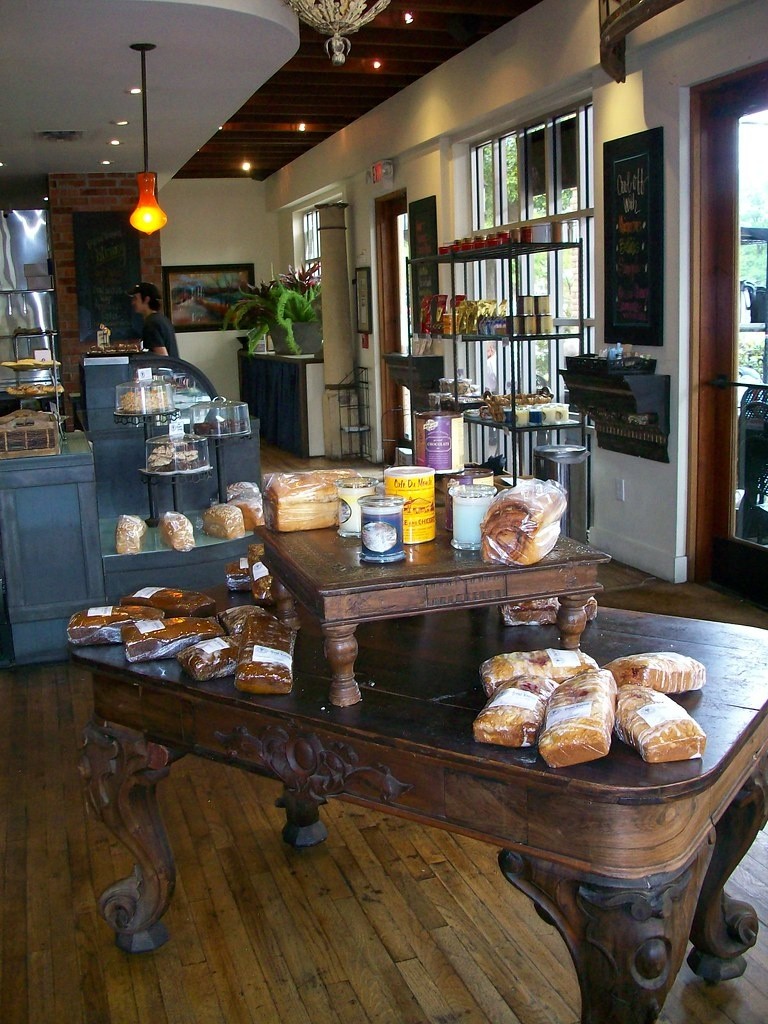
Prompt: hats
<box><xmin>125</xmin><ymin>282</ymin><xmax>162</xmax><ymax>301</ymax></box>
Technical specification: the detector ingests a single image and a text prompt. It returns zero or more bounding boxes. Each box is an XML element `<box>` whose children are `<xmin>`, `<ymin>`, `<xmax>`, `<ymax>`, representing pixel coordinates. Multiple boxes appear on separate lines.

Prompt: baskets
<box><xmin>484</xmin><ymin>387</ymin><xmax>554</xmax><ymax>422</ymax></box>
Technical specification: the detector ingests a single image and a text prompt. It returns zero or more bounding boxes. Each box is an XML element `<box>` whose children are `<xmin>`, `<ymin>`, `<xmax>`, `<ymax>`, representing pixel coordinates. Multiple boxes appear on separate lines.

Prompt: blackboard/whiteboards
<box><xmin>72</xmin><ymin>209</ymin><xmax>144</xmax><ymax>340</ymax></box>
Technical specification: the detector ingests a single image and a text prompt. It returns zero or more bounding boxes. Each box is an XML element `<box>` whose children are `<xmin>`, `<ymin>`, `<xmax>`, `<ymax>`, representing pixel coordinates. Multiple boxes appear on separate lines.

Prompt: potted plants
<box><xmin>224</xmin><ymin>256</ymin><xmax>323</xmax><ymax>355</ymax></box>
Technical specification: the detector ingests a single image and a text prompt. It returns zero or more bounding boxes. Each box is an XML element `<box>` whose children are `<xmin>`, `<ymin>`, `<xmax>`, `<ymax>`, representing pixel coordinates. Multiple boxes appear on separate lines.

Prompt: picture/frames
<box><xmin>354</xmin><ymin>267</ymin><xmax>372</xmax><ymax>335</ymax></box>
<box><xmin>602</xmin><ymin>126</ymin><xmax>665</xmax><ymax>347</ymax></box>
<box><xmin>161</xmin><ymin>262</ymin><xmax>258</xmax><ymax>334</ymax></box>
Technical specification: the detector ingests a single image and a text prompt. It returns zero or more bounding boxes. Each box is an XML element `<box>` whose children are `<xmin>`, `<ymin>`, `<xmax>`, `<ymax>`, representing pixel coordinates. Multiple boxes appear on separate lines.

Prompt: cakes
<box><xmin>194</xmin><ymin>417</ymin><xmax>248</xmax><ymax>435</ymax></box>
<box><xmin>119</xmin><ymin>388</ymin><xmax>170</xmax><ymax>414</ymax></box>
<box><xmin>148</xmin><ymin>442</ymin><xmax>206</xmax><ymax>472</ymax></box>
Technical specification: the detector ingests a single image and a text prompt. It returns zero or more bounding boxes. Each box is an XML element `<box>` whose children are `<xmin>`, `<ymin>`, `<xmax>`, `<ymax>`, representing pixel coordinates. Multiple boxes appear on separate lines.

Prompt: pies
<box><xmin>1</xmin><ymin>359</ymin><xmax>62</xmax><ymax>366</ymax></box>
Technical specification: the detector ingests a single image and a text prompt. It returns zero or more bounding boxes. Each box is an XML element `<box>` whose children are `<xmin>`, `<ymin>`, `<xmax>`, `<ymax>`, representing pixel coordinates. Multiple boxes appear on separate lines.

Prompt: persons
<box><xmin>124</xmin><ymin>282</ymin><xmax>179</xmax><ymax>358</ymax></box>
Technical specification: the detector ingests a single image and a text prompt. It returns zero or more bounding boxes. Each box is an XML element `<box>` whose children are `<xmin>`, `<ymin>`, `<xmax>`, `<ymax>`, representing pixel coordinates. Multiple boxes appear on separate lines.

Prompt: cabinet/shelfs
<box><xmin>237</xmin><ymin>349</ymin><xmax>327</xmax><ymax>460</ymax></box>
<box><xmin>406</xmin><ymin>238</ymin><xmax>583</xmax><ymax>488</ymax></box>
<box><xmin>338</xmin><ymin>367</ymin><xmax>372</xmax><ymax>465</ymax></box>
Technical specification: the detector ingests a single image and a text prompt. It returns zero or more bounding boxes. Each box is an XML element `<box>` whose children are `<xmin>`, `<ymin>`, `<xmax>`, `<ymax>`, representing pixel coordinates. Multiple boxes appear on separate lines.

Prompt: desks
<box><xmin>380</xmin><ymin>351</ymin><xmax>444</xmax><ymax>467</ymax></box>
<box><xmin>66</xmin><ymin>574</ymin><xmax>768</xmax><ymax>1024</ymax></box>
<box><xmin>253</xmin><ymin>524</ymin><xmax>611</xmax><ymax>706</ymax></box>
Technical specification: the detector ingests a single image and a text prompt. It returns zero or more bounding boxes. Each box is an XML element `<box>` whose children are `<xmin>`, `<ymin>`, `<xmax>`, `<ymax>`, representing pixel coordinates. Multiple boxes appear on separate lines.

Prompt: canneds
<box><xmin>384</xmin><ymin>411</ymin><xmax>495</xmax><ymax>544</ymax></box>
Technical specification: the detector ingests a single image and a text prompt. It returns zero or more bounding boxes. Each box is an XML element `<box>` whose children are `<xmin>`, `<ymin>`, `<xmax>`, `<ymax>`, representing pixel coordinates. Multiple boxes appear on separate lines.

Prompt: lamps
<box><xmin>129</xmin><ymin>42</ymin><xmax>167</xmax><ymax>238</ymax></box>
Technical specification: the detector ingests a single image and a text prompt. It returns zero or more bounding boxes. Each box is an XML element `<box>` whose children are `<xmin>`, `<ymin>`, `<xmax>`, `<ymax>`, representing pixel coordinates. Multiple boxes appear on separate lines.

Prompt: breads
<box><xmin>162</xmin><ymin>468</ymin><xmax>362</xmax><ymax>552</ymax></box>
<box><xmin>5</xmin><ymin>383</ymin><xmax>65</xmax><ymax>395</ymax></box>
<box><xmin>480</xmin><ymin>487</ymin><xmax>568</xmax><ymax>564</ymax></box>
<box><xmin>115</xmin><ymin>514</ymin><xmax>144</xmax><ymax>555</ymax></box>
<box><xmin>500</xmin><ymin>597</ymin><xmax>598</xmax><ymax>627</ymax></box>
<box><xmin>472</xmin><ymin>648</ymin><xmax>707</xmax><ymax>768</ymax></box>
<box><xmin>67</xmin><ymin>546</ymin><xmax>296</xmax><ymax>694</ymax></box>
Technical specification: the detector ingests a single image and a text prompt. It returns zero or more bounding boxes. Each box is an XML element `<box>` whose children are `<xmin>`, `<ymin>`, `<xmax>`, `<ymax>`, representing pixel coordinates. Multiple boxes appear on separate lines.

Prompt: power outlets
<box><xmin>615</xmin><ymin>479</ymin><xmax>625</xmax><ymax>502</ymax></box>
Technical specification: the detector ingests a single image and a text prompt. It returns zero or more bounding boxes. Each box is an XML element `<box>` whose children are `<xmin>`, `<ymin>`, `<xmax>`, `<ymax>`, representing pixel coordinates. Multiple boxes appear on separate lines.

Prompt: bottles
<box><xmin>337</xmin><ymin>476</ymin><xmax>379</xmax><ymax>537</ymax></box>
<box><xmin>358</xmin><ymin>495</ymin><xmax>407</xmax><ymax>563</ymax></box>
<box><xmin>447</xmin><ymin>484</ymin><xmax>497</xmax><ymax>551</ymax></box>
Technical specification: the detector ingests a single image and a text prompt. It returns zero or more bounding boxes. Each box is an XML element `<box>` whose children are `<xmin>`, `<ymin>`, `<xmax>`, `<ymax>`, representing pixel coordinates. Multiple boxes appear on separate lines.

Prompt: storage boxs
<box><xmin>23</xmin><ymin>264</ymin><xmax>52</xmax><ymax>291</ymax></box>
<box><xmin>0</xmin><ymin>408</ymin><xmax>60</xmax><ymax>460</ymax></box>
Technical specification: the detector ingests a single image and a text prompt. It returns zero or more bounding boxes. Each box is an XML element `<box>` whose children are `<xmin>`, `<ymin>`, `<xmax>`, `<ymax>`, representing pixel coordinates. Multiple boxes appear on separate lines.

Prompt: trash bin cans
<box><xmin>534</xmin><ymin>443</ymin><xmax>590</xmax><ymax>547</ymax></box>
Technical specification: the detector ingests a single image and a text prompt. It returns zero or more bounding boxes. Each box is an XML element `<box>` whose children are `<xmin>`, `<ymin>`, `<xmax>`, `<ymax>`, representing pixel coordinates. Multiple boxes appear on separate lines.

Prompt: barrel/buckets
<box><xmin>445</xmin><ymin>469</ymin><xmax>496</xmax><ymax>531</ymax></box>
<box><xmin>505</xmin><ymin>296</ymin><xmax>554</xmax><ymax>334</ymax></box>
<box><xmin>412</xmin><ymin>409</ymin><xmax>465</xmax><ymax>474</ymax></box>
<box><xmin>385</xmin><ymin>467</ymin><xmax>438</xmax><ymax>545</ymax></box>
